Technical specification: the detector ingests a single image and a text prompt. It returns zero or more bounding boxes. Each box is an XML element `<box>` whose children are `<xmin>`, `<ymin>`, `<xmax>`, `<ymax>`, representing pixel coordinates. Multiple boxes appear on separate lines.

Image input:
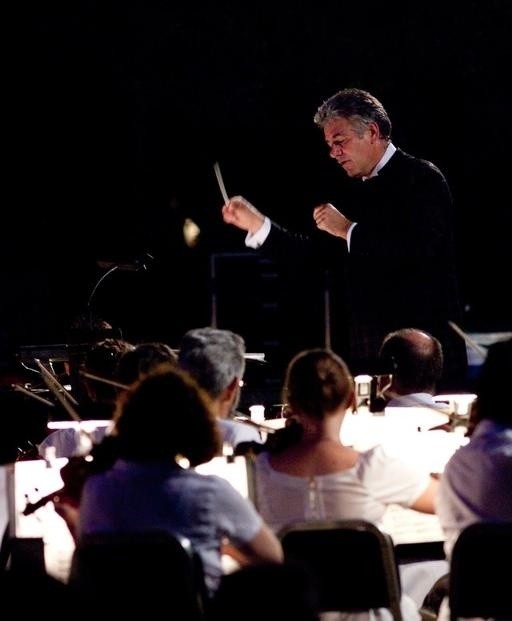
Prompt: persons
<box><xmin>427</xmin><ymin>333</ymin><xmax>512</xmax><ymax>621</ymax></box>
<box><xmin>376</xmin><ymin>328</ymin><xmax>469</xmax><ymax>472</ymax></box>
<box><xmin>47</xmin><ymin>362</ymin><xmax>287</xmax><ymax>621</ymax></box>
<box><xmin>82</xmin><ymin>338</ymin><xmax>132</xmax><ymax>400</ymax></box>
<box><xmin>221</xmin><ymin>86</ymin><xmax>454</xmax><ymax>374</ymax></box>
<box><xmin>182</xmin><ymin>325</ymin><xmax>261</xmax><ymax>449</ymax></box>
<box><xmin>118</xmin><ymin>342</ymin><xmax>177</xmax><ymax>381</ymax></box>
<box><xmin>234</xmin><ymin>349</ymin><xmax>444</xmax><ymax>621</ymax></box>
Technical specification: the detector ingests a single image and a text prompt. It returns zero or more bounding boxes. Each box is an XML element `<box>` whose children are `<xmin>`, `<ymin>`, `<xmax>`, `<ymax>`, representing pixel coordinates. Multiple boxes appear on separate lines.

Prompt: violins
<box><xmin>226</xmin><ymin>422</ymin><xmax>303</xmax><ymax>466</ymax></box>
<box><xmin>429</xmin><ymin>404</ymin><xmax>478</xmax><ymax>437</ymax></box>
<box><xmin>23</xmin><ymin>433</ymin><xmax>127</xmax><ymax>519</ymax></box>
